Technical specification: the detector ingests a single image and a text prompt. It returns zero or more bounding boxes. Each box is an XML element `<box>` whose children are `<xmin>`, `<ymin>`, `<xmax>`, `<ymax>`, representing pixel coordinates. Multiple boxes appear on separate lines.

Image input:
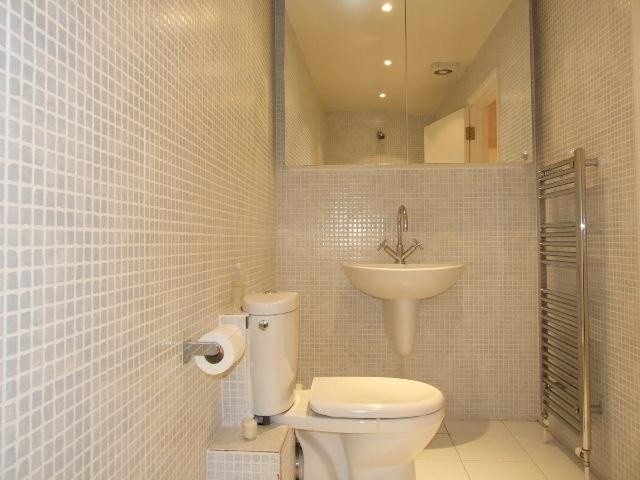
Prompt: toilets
<box><xmin>241</xmin><ymin>290</ymin><xmax>446</xmax><ymax>480</ymax></box>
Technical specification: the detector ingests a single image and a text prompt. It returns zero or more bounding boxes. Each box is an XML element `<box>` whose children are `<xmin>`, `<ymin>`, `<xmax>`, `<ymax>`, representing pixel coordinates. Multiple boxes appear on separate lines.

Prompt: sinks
<box><xmin>342</xmin><ymin>262</ymin><xmax>465</xmax><ymax>299</ymax></box>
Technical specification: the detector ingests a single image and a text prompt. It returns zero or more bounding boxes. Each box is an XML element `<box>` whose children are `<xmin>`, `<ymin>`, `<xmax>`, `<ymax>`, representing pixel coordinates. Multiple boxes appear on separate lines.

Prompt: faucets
<box><xmin>396</xmin><ymin>205</ymin><xmax>408</xmax><ymax>255</ymax></box>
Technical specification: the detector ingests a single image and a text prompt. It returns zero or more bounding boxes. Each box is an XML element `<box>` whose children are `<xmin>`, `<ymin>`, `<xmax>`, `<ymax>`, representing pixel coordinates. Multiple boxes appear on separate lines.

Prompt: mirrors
<box><xmin>283</xmin><ymin>0</ymin><xmax>535</xmax><ymax>167</ymax></box>
